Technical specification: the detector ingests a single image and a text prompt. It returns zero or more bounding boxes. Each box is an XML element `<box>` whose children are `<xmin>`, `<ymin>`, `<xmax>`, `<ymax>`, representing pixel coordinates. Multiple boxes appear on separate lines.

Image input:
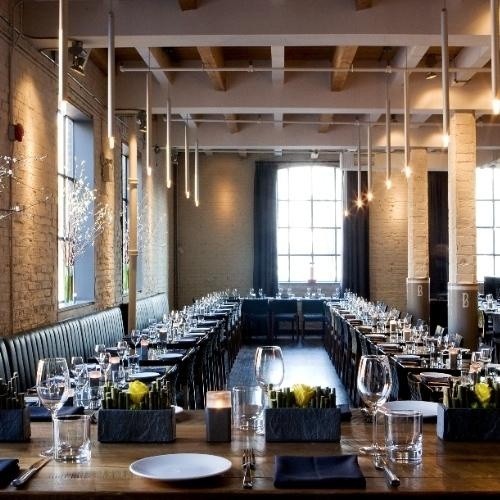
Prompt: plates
<box><xmin>377</xmin><ymin>400</ymin><xmax>442</xmax><ymax>419</ymax></box>
<box><xmin>129</xmin><ymin>453</ymin><xmax>233</xmax><ymax>482</ymax></box>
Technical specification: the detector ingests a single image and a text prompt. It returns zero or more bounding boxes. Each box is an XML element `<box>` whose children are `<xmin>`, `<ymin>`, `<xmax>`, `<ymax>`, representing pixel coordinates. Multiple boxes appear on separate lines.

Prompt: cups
<box><xmin>247</xmin><ymin>288</ymin><xmax>322</xmax><ymax>299</ymax></box>
<box><xmin>67</xmin><ymin>287</ymin><xmax>238</xmax><ymax>413</ymax></box>
<box><xmin>386</xmin><ymin>411</ymin><xmax>423</xmax><ymax>466</ymax></box>
<box><xmin>231</xmin><ymin>386</ymin><xmax>267</xmax><ymax>458</ymax></box>
<box><xmin>52</xmin><ymin>414</ymin><xmax>91</xmax><ymax>466</ymax></box>
<box><xmin>332</xmin><ymin>288</ymin><xmax>496</xmax><ymax>388</ymax></box>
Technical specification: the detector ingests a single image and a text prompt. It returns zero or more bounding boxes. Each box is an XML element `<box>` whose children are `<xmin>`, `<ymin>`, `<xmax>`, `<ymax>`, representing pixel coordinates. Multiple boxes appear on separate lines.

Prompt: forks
<box><xmin>242</xmin><ymin>448</ymin><xmax>256</xmax><ymax>487</ymax></box>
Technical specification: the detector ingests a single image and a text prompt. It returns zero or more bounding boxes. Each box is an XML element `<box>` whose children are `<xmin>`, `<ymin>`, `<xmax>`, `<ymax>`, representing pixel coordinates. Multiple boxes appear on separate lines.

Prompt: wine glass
<box><xmin>255</xmin><ymin>345</ymin><xmax>284</xmax><ymax>434</ymax></box>
<box><xmin>357</xmin><ymin>355</ymin><xmax>393</xmax><ymax>455</ymax></box>
<box><xmin>38</xmin><ymin>357</ymin><xmax>70</xmax><ymax>458</ymax></box>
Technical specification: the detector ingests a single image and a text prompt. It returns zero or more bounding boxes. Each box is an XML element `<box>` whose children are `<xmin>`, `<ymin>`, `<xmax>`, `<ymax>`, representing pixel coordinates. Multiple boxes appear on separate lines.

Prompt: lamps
<box><xmin>340</xmin><ymin>1</ymin><xmax>500</xmax><ymax>218</ymax></box>
<box><xmin>56</xmin><ymin>0</ymin><xmax>201</xmax><ymax>209</ymax></box>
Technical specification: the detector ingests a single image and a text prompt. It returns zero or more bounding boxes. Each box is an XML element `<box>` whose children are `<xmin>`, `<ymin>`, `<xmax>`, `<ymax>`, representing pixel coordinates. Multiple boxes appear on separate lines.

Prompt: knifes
<box><xmin>12</xmin><ymin>456</ymin><xmax>52</xmax><ymax>489</ymax></box>
<box><xmin>372</xmin><ymin>451</ymin><xmax>399</xmax><ymax>488</ymax></box>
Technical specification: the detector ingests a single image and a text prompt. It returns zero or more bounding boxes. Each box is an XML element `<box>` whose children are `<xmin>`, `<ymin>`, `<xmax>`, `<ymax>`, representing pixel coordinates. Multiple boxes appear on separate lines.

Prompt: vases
<box><xmin>119</xmin><ymin>262</ymin><xmax>133</xmax><ymax>295</ymax></box>
<box><xmin>60</xmin><ymin>263</ymin><xmax>77</xmax><ymax>305</ymax></box>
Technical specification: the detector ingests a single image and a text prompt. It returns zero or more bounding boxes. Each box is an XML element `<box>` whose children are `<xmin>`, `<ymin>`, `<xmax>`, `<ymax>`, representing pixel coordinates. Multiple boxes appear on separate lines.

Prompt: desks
<box><xmin>241</xmin><ymin>290</ymin><xmax>324</xmax><ymax>344</ymax></box>
<box><xmin>0</xmin><ymin>398</ymin><xmax>500</xmax><ymax>498</ymax></box>
<box><xmin>324</xmin><ymin>291</ymin><xmax>499</xmax><ymax>401</ymax></box>
<box><xmin>1</xmin><ymin>291</ymin><xmax>244</xmax><ymax>410</ymax></box>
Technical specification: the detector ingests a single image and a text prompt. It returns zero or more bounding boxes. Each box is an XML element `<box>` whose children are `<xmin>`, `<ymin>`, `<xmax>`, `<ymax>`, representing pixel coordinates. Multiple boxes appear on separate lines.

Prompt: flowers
<box><xmin>119</xmin><ymin>199</ymin><xmax>150</xmax><ymax>265</ymax></box>
<box><xmin>55</xmin><ymin>151</ymin><xmax>114</xmax><ymax>267</ymax></box>
